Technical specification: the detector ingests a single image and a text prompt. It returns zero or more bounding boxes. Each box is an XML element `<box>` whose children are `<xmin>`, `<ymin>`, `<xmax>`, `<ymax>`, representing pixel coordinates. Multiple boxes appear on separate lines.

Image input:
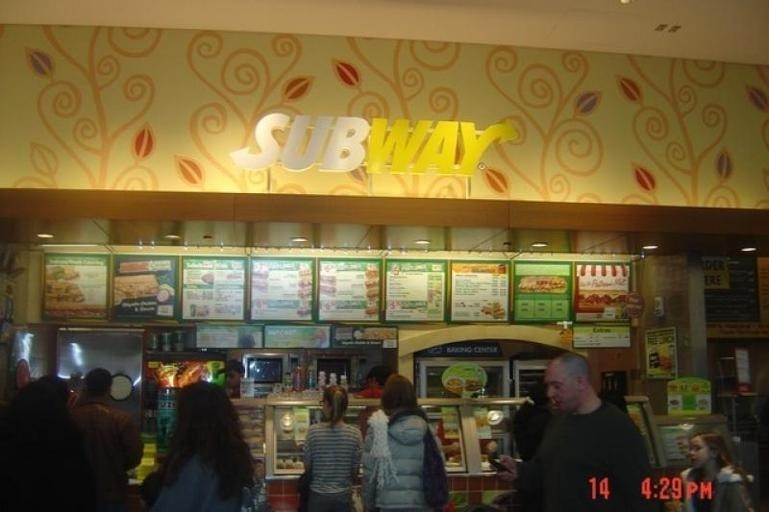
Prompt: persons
<box><xmin>301</xmin><ymin>385</ymin><xmax>365</xmax><ymax>511</ymax></box>
<box><xmin>216</xmin><ymin>359</ymin><xmax>246</xmax><ymax>398</ymax></box>
<box><xmin>0</xmin><ymin>374</ymin><xmax>97</xmax><ymax>511</ymax></box>
<box><xmin>138</xmin><ymin>380</ymin><xmax>258</xmax><ymax>512</ymax></box>
<box><xmin>360</xmin><ymin>374</ymin><xmax>448</xmax><ymax>511</ymax></box>
<box><xmin>512</xmin><ymin>380</ymin><xmax>552</xmax><ymax>461</ymax></box>
<box><xmin>679</xmin><ymin>430</ymin><xmax>759</xmax><ymax>511</ymax></box>
<box><xmin>70</xmin><ymin>367</ymin><xmax>144</xmax><ymax>511</ymax></box>
<box><xmin>487</xmin><ymin>352</ymin><xmax>666</xmax><ymax>512</ymax></box>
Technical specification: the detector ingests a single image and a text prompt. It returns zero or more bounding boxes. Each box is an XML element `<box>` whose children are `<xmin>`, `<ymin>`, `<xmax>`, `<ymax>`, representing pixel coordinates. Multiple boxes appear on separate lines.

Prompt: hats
<box><xmin>217</xmin><ymin>359</ymin><xmax>249</xmax><ymax>378</ymax></box>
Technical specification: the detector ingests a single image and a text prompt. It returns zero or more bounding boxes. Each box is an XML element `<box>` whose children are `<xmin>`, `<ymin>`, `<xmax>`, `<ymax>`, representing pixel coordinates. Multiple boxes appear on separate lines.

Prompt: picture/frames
<box><xmin>645</xmin><ymin>327</ymin><xmax>677</xmax><ymax>381</ymax></box>
<box><xmin>704</xmin><ymin>255</ymin><xmax>768</xmax><ymax>341</ymax></box>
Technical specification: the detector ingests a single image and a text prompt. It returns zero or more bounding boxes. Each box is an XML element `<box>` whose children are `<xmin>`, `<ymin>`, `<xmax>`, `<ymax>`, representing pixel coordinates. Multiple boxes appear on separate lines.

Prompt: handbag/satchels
<box><xmin>239</xmin><ymin>477</ymin><xmax>270</xmax><ymax>511</ymax></box>
<box><xmin>421</xmin><ymin>435</ymin><xmax>449</xmax><ymax>508</ymax></box>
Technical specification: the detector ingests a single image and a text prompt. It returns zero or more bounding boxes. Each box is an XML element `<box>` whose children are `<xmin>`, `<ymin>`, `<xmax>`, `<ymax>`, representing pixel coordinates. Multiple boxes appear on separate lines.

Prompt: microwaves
<box><xmin>241</xmin><ymin>351</ymin><xmax>300</xmax><ymax>386</ymax></box>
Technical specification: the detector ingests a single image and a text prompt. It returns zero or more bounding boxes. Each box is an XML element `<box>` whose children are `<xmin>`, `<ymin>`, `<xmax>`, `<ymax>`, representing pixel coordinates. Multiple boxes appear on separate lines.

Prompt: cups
<box><xmin>444</xmin><ymin>376</ymin><xmax>465</xmax><ymax>397</ymax></box>
<box><xmin>145</xmin><ymin>330</ymin><xmax>186</xmax><ymax>352</ymax></box>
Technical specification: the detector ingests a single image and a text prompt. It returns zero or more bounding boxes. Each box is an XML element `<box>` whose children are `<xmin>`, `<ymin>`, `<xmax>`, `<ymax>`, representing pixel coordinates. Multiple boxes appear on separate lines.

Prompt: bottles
<box><xmin>284</xmin><ymin>364</ymin><xmax>350</xmax><ymax>396</ymax></box>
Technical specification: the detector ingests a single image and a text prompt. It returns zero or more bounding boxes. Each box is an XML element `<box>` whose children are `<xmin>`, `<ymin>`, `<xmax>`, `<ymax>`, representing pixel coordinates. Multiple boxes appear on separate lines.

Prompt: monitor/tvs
<box><xmin>313</xmin><ymin>356</ymin><xmax>350</xmax><ymax>389</ymax></box>
<box><xmin>242</xmin><ymin>353</ymin><xmax>288</xmax><ymax>394</ymax></box>
